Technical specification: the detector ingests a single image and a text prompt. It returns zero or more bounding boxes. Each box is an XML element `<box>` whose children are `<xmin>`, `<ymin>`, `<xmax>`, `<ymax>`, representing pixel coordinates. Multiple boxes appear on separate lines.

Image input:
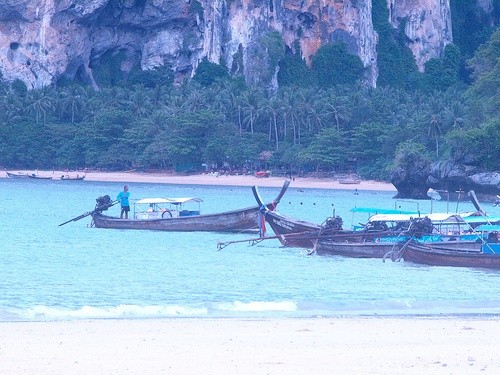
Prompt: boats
<box><xmin>89</xmin><ymin>178</ymin><xmax>293</xmax><ymax>234</ymax></box>
<box><xmin>6</xmin><ymin>171</ymin><xmax>28</xmax><ymax>178</ymax></box>
<box><xmin>249</xmin><ymin>186</ymin><xmax>500</xmax><ymax>268</ymax></box>
<box><xmin>26</xmin><ymin>173</ymin><xmax>52</xmax><ymax>179</ymax></box>
<box><xmin>61</xmin><ymin>175</ymin><xmax>86</xmax><ymax>180</ymax></box>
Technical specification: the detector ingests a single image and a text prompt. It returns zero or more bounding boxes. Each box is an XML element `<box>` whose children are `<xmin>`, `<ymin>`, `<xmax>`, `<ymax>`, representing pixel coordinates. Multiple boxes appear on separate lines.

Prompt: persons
<box><xmin>437</xmin><ymin>229</ymin><xmax>480</xmax><ymax>235</ymax></box>
<box><xmin>395</xmin><ymin>216</ymin><xmax>432</xmax><ymax>233</ymax></box>
<box><xmin>327</xmin><ymin>216</ymin><xmax>343</xmax><ymax>230</ymax></box>
<box><xmin>302</xmin><ymin>168</ymin><xmax>358</xmax><ymax>178</ymax></box>
<box><xmin>365</xmin><ymin>221</ymin><xmax>387</xmax><ymax>233</ymax></box>
<box><xmin>186</xmin><ymin>166</ymin><xmax>270</xmax><ymax>175</ymax></box>
<box><xmin>117</xmin><ymin>186</ymin><xmax>130</xmax><ymax>219</ymax></box>
<box><xmin>488</xmin><ymin>233</ymin><xmax>500</xmax><ymax>243</ymax></box>
